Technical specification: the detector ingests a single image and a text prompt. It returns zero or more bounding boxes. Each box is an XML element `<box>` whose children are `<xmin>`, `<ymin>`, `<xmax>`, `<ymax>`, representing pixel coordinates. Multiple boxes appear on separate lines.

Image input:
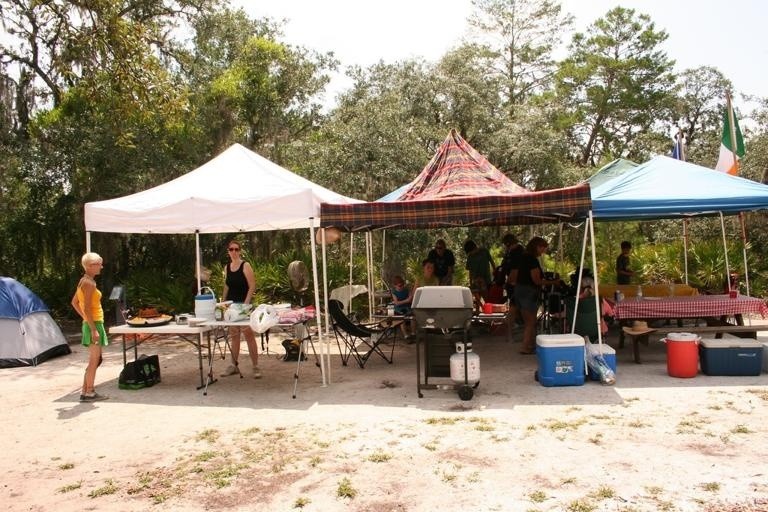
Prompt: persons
<box><xmin>615</xmin><ymin>241</ymin><xmax>636</xmax><ymax>285</ymax></box>
<box><xmin>220</xmin><ymin>239</ymin><xmax>262</xmax><ymax>379</ymax></box>
<box><xmin>71</xmin><ymin>251</ymin><xmax>109</xmax><ymax>402</ymax></box>
<box><xmin>389</xmin><ymin>233</ymin><xmax>561</xmax><ymax>354</ymax></box>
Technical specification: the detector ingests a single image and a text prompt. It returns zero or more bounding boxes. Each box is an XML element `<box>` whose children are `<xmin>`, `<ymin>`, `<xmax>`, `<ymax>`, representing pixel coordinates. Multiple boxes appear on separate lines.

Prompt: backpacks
<box><xmin>281</xmin><ymin>338</ymin><xmax>304</xmax><ymax>362</ymax></box>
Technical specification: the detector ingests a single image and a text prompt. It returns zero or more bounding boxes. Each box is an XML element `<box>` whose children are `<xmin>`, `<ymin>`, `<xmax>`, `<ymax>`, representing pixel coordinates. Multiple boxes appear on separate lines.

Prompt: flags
<box><xmin>715</xmin><ymin>96</ymin><xmax>744</xmax><ymax>175</ymax></box>
<box><xmin>670</xmin><ymin>137</ymin><xmax>680</xmax><ymax>160</ymax></box>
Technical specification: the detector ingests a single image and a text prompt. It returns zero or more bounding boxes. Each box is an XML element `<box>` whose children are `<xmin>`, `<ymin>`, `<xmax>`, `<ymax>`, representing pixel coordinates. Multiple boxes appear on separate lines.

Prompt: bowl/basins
<box><xmin>175</xmin><ymin>312</ymin><xmax>196</xmax><ymax>325</ymax></box>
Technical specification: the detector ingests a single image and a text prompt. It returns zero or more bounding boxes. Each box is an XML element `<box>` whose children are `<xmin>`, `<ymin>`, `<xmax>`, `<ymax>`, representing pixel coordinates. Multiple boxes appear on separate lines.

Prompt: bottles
<box><xmin>214</xmin><ymin>300</ymin><xmax>234</xmax><ymax>322</ymax></box>
<box><xmin>636</xmin><ymin>284</ymin><xmax>642</xmax><ymax>302</ymax></box>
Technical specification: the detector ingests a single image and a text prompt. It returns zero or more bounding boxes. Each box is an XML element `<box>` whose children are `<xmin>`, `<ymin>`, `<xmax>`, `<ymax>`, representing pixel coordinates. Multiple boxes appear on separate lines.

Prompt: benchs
<box><xmin>624</xmin><ymin>324</ymin><xmax>768</xmax><ymax>364</ymax></box>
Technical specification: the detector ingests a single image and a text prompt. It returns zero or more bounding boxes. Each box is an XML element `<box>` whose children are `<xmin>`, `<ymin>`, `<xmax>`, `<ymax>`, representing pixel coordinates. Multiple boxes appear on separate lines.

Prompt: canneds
<box><xmin>614</xmin><ymin>289</ymin><xmax>622</xmax><ymax>302</ymax></box>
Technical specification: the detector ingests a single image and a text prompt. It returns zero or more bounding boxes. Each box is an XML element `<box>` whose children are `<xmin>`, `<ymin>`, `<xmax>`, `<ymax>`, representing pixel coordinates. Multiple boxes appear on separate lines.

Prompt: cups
<box><xmin>483</xmin><ymin>303</ymin><xmax>493</xmax><ymax>314</ymax></box>
<box><xmin>729</xmin><ymin>289</ymin><xmax>738</xmax><ymax>298</ymax></box>
<box><xmin>387</xmin><ymin>304</ymin><xmax>395</xmax><ymax>316</ymax></box>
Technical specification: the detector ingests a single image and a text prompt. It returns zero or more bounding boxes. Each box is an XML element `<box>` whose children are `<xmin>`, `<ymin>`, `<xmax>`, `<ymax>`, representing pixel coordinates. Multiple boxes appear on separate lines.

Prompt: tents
<box><xmin>0</xmin><ymin>274</ymin><xmax>72</xmax><ymax>367</ymax></box>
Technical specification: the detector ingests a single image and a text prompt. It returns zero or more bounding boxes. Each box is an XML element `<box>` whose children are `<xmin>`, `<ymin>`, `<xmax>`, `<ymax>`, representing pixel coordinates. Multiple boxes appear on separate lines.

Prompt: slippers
<box><xmin>519</xmin><ymin>344</ymin><xmax>536</xmax><ymax>355</ymax></box>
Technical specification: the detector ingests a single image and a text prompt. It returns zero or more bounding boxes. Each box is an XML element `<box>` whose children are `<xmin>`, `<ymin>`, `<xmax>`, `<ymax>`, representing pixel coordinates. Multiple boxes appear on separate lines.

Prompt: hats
<box><xmin>622</xmin><ymin>320</ymin><xmax>658</xmax><ymax>336</ymax></box>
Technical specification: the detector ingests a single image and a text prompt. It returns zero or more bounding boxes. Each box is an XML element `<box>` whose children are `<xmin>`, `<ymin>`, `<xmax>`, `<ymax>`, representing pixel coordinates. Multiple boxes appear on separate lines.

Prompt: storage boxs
<box><xmin>700</xmin><ymin>338</ymin><xmax>763</xmax><ymax>376</ymax></box>
<box><xmin>587</xmin><ymin>342</ymin><xmax>617</xmax><ymax>382</ymax></box>
<box><xmin>535</xmin><ymin>333</ymin><xmax>587</xmax><ymax>387</ymax></box>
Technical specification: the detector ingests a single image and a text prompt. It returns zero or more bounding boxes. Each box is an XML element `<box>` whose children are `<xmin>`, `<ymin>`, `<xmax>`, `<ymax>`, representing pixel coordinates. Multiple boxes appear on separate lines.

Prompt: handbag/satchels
<box><xmin>118</xmin><ymin>354</ymin><xmax>161</xmax><ymax>390</ymax></box>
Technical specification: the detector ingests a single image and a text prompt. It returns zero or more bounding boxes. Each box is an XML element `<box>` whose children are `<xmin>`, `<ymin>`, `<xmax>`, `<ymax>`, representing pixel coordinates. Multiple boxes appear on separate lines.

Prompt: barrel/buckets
<box><xmin>194</xmin><ymin>286</ymin><xmax>218</xmax><ymax>322</ymax></box>
<box><xmin>661</xmin><ymin>329</ymin><xmax>702</xmax><ymax>378</ymax></box>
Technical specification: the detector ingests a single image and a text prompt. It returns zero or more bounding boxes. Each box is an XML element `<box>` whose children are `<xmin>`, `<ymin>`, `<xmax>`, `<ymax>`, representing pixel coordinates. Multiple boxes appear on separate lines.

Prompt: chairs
<box><xmin>566</xmin><ymin>294</ymin><xmax>603</xmax><ymax>344</ymax></box>
<box><xmin>328</xmin><ymin>299</ymin><xmax>404</xmax><ymax>368</ymax></box>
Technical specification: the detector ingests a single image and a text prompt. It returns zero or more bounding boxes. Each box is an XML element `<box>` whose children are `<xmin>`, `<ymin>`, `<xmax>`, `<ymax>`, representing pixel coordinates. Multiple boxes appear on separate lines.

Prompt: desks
<box><xmin>602</xmin><ymin>294</ymin><xmax>768</xmax><ymax>346</ymax></box>
<box><xmin>370</xmin><ymin>314</ymin><xmax>508</xmax><ymax>362</ymax></box>
<box><xmin>107</xmin><ymin>324</ymin><xmax>218</xmax><ymax>390</ymax></box>
<box><xmin>196</xmin><ymin>319</ymin><xmax>324</xmax><ymax>399</ymax></box>
<box><xmin>593</xmin><ymin>282</ymin><xmax>699</xmax><ymax>327</ymax></box>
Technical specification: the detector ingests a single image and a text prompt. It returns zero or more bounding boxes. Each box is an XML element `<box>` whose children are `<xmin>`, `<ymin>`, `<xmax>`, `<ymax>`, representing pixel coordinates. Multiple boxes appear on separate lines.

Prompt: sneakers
<box><xmin>252</xmin><ymin>366</ymin><xmax>262</xmax><ymax>379</ymax></box>
<box><xmin>219</xmin><ymin>365</ymin><xmax>239</xmax><ymax>376</ymax></box>
<box><xmin>405</xmin><ymin>334</ymin><xmax>420</xmax><ymax>344</ymax></box>
<box><xmin>79</xmin><ymin>394</ymin><xmax>109</xmax><ymax>402</ymax></box>
